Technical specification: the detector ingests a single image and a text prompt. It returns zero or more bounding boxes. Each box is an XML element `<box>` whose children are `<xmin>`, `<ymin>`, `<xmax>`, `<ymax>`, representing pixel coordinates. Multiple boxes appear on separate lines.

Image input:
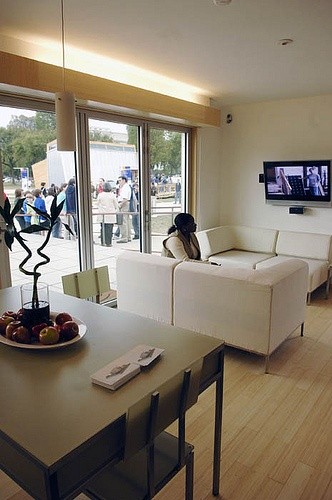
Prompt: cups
<box><xmin>21</xmin><ymin>282</ymin><xmax>48</xmax><ymax>321</ymax></box>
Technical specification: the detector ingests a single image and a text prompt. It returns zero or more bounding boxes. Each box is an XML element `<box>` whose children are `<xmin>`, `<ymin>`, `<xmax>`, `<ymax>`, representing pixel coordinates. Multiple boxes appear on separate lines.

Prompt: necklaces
<box><xmin>27</xmin><ymin>195</ymin><xmax>40</xmax><ymax>226</ymax></box>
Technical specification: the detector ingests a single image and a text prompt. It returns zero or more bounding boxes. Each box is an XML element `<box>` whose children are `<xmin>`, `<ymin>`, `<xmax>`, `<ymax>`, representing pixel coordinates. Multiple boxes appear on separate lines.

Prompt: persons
<box><xmin>298</xmin><ymin>168</ymin><xmax>321</xmax><ymax>195</ymax></box>
<box><xmin>162</xmin><ymin>213</ymin><xmax>221</xmax><ymax>266</ymax></box>
<box><xmin>51</xmin><ymin>183</ymin><xmax>55</xmax><ymax>187</ymax></box>
<box><xmin>162</xmin><ymin>175</ymin><xmax>165</xmax><ymax>179</ymax></box>
<box><xmin>165</xmin><ymin>176</ymin><xmax>168</xmax><ymax>183</ymax></box>
<box><xmin>97</xmin><ymin>177</ymin><xmax>105</xmax><ymax>193</ymax></box>
<box><xmin>175</xmin><ymin>179</ymin><xmax>181</xmax><ymax>203</ymax></box>
<box><xmin>45</xmin><ymin>188</ymin><xmax>55</xmax><ymax>227</ymax></box>
<box><xmin>113</xmin><ymin>180</ymin><xmax>120</xmax><ymax>240</ymax></box>
<box><xmin>277</xmin><ymin>168</ymin><xmax>292</xmax><ymax>195</ymax></box>
<box><xmin>133</xmin><ymin>184</ymin><xmax>140</xmax><ymax>238</ymax></box>
<box><xmin>66</xmin><ymin>178</ymin><xmax>79</xmax><ymax>239</ymax></box>
<box><xmin>57</xmin><ymin>183</ymin><xmax>68</xmax><ymax>236</ymax></box>
<box><xmin>150</xmin><ymin>182</ymin><xmax>156</xmax><ymax>195</ymax></box>
<box><xmin>155</xmin><ymin>177</ymin><xmax>159</xmax><ymax>183</ymax></box>
<box><xmin>117</xmin><ymin>176</ymin><xmax>133</xmax><ymax>243</ymax></box>
<box><xmin>40</xmin><ymin>182</ymin><xmax>48</xmax><ymax>197</ymax></box>
<box><xmin>23</xmin><ymin>190</ymin><xmax>35</xmax><ymax>228</ymax></box>
<box><xmin>52</xmin><ymin>186</ymin><xmax>64</xmax><ymax>239</ymax></box>
<box><xmin>33</xmin><ymin>188</ymin><xmax>47</xmax><ymax>224</ymax></box>
<box><xmin>97</xmin><ymin>183</ymin><xmax>120</xmax><ymax>247</ymax></box>
<box><xmin>13</xmin><ymin>188</ymin><xmax>26</xmax><ymax>231</ymax></box>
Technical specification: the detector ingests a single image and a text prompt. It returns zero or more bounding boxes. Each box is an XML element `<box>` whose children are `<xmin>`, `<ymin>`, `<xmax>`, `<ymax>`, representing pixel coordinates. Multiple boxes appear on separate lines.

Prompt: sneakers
<box><xmin>106</xmin><ymin>244</ymin><xmax>113</xmax><ymax>247</ymax></box>
<box><xmin>117</xmin><ymin>238</ymin><xmax>128</xmax><ymax>243</ymax></box>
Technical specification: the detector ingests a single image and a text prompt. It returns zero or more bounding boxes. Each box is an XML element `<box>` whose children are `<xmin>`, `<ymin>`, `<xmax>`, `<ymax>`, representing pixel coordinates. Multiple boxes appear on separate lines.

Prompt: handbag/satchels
<box><xmin>117</xmin><ymin>210</ymin><xmax>123</xmax><ymax>225</ymax></box>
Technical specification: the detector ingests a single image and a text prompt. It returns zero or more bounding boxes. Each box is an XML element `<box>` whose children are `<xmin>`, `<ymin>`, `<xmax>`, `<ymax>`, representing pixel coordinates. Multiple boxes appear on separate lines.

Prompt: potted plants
<box><xmin>0</xmin><ymin>195</ymin><xmax>66</xmax><ymax>322</ymax></box>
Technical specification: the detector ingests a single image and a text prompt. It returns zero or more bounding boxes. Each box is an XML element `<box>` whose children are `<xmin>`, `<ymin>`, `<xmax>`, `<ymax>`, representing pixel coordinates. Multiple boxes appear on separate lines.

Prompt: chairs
<box><xmin>61</xmin><ymin>265</ymin><xmax>117</xmax><ymax>306</ymax></box>
<box><xmin>83</xmin><ymin>358</ymin><xmax>204</xmax><ymax>500</ymax></box>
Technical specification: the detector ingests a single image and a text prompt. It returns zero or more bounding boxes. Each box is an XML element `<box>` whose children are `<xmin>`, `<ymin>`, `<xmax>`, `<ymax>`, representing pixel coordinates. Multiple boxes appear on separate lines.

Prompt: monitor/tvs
<box><xmin>263</xmin><ymin>160</ymin><xmax>332</xmax><ymax>208</ymax></box>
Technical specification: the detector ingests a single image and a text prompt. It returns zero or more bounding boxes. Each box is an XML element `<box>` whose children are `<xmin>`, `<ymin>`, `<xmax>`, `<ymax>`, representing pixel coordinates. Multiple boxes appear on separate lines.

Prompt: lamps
<box><xmin>54</xmin><ymin>0</ymin><xmax>76</xmax><ymax>150</ymax></box>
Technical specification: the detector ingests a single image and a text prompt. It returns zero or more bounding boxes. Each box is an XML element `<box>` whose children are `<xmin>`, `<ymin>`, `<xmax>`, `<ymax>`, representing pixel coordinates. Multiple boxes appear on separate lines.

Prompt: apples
<box><xmin>61</xmin><ymin>320</ymin><xmax>79</xmax><ymax>339</ymax></box>
<box><xmin>0</xmin><ymin>315</ymin><xmax>15</xmax><ymax>333</ymax></box>
<box><xmin>16</xmin><ymin>314</ymin><xmax>23</xmax><ymax>321</ymax></box>
<box><xmin>16</xmin><ymin>308</ymin><xmax>23</xmax><ymax>315</ymax></box>
<box><xmin>3</xmin><ymin>311</ymin><xmax>16</xmax><ymax>318</ymax></box>
<box><xmin>55</xmin><ymin>312</ymin><xmax>73</xmax><ymax>324</ymax></box>
<box><xmin>11</xmin><ymin>325</ymin><xmax>30</xmax><ymax>341</ymax></box>
<box><xmin>32</xmin><ymin>322</ymin><xmax>47</xmax><ymax>334</ymax></box>
<box><xmin>5</xmin><ymin>321</ymin><xmax>24</xmax><ymax>338</ymax></box>
<box><xmin>38</xmin><ymin>326</ymin><xmax>61</xmax><ymax>344</ymax></box>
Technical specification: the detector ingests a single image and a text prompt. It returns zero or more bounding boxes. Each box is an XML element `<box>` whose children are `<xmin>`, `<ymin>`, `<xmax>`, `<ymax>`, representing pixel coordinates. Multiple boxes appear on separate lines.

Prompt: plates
<box><xmin>0</xmin><ymin>310</ymin><xmax>87</xmax><ymax>350</ymax></box>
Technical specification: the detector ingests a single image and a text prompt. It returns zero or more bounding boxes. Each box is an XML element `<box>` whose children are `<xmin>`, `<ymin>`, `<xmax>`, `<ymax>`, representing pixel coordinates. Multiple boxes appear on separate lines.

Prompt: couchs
<box><xmin>194</xmin><ymin>225</ymin><xmax>332</xmax><ymax>305</ymax></box>
<box><xmin>116</xmin><ymin>248</ymin><xmax>308</xmax><ymax>373</ymax></box>
